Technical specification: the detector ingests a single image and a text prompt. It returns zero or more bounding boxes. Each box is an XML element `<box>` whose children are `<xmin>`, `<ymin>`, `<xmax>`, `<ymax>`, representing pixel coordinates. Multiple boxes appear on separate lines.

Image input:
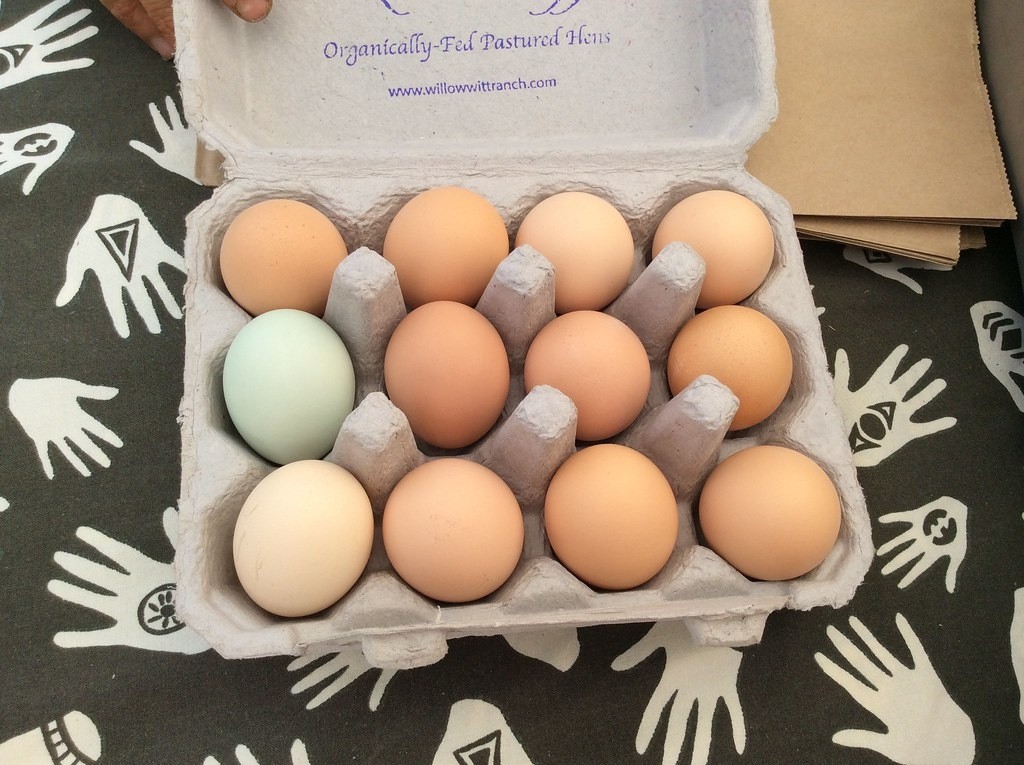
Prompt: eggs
<box><xmin>223</xmin><ymin>309</ymin><xmax>355</xmax><ymax>466</ymax></box>
<box><xmin>544</xmin><ymin>444</ymin><xmax>678</xmax><ymax>591</ymax></box>
<box><xmin>383</xmin><ymin>186</ymin><xmax>509</xmax><ymax>310</ymax></box>
<box><xmin>514</xmin><ymin>191</ymin><xmax>634</xmax><ymax>316</ymax></box>
<box><xmin>383</xmin><ymin>458</ymin><xmax>524</xmax><ymax>603</ymax></box>
<box><xmin>699</xmin><ymin>445</ymin><xmax>841</xmax><ymax>581</ymax></box>
<box><xmin>383</xmin><ymin>301</ymin><xmax>510</xmax><ymax>450</ymax></box>
<box><xmin>232</xmin><ymin>457</ymin><xmax>374</xmax><ymax>617</ymax></box>
<box><xmin>524</xmin><ymin>311</ymin><xmax>651</xmax><ymax>441</ymax></box>
<box><xmin>667</xmin><ymin>304</ymin><xmax>793</xmax><ymax>432</ymax></box>
<box><xmin>652</xmin><ymin>189</ymin><xmax>774</xmax><ymax>311</ymax></box>
<box><xmin>219</xmin><ymin>198</ymin><xmax>348</xmax><ymax>318</ymax></box>
